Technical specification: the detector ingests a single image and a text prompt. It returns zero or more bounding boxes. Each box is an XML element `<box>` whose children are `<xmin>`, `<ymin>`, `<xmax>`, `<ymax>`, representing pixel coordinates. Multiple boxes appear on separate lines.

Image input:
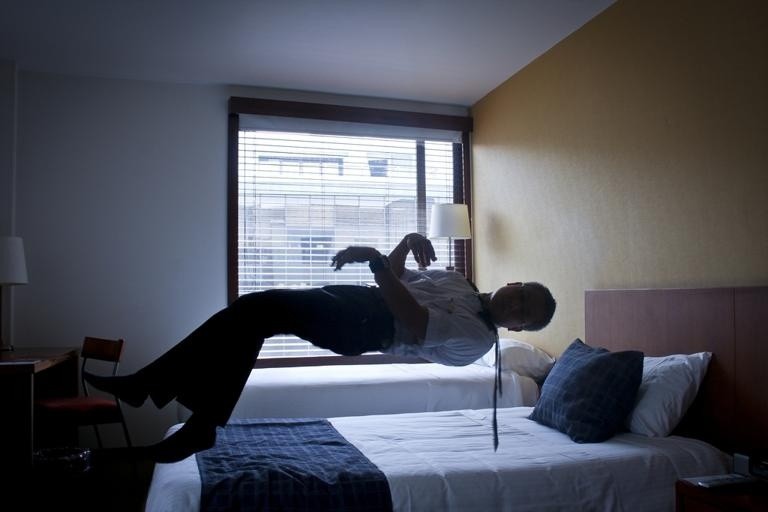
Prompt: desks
<box><xmin>0</xmin><ymin>344</ymin><xmax>77</xmax><ymax>464</ymax></box>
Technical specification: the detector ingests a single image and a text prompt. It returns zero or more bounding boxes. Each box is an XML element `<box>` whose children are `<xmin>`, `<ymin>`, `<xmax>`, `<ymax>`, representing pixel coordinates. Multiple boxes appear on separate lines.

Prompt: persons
<box><xmin>84</xmin><ymin>234</ymin><xmax>558</xmax><ymax>465</ymax></box>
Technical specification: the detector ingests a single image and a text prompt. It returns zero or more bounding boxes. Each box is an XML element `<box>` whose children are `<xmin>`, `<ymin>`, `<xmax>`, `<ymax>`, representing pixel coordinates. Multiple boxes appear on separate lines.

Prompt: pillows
<box><xmin>483</xmin><ymin>338</ymin><xmax>712</xmax><ymax>441</ymax></box>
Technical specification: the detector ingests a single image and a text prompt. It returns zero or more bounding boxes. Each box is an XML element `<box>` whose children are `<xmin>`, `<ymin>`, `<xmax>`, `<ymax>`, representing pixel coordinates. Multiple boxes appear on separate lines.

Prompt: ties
<box><xmin>482</xmin><ymin>315</ymin><xmax>503</xmax><ymax>452</ymax></box>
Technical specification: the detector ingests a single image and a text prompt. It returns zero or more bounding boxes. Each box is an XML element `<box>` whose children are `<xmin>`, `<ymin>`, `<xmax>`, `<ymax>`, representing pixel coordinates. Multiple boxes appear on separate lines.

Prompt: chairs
<box><xmin>33</xmin><ymin>337</ymin><xmax>137</xmax><ymax>473</ymax></box>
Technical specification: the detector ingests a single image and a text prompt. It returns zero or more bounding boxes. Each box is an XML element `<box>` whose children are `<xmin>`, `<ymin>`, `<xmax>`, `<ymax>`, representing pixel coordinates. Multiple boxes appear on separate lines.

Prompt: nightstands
<box><xmin>675</xmin><ymin>472</ymin><xmax>768</xmax><ymax>512</ymax></box>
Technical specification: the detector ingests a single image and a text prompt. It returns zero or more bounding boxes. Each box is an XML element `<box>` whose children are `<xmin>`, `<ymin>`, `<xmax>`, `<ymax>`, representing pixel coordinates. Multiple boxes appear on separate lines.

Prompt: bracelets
<box><xmin>368</xmin><ymin>254</ymin><xmax>392</xmax><ymax>272</ymax></box>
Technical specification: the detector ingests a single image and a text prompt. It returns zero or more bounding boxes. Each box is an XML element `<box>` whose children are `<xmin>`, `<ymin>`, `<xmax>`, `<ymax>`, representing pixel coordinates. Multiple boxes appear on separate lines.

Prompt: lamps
<box><xmin>1</xmin><ymin>235</ymin><xmax>30</xmax><ymax>349</ymax></box>
<box><xmin>429</xmin><ymin>203</ymin><xmax>472</xmax><ymax>270</ymax></box>
